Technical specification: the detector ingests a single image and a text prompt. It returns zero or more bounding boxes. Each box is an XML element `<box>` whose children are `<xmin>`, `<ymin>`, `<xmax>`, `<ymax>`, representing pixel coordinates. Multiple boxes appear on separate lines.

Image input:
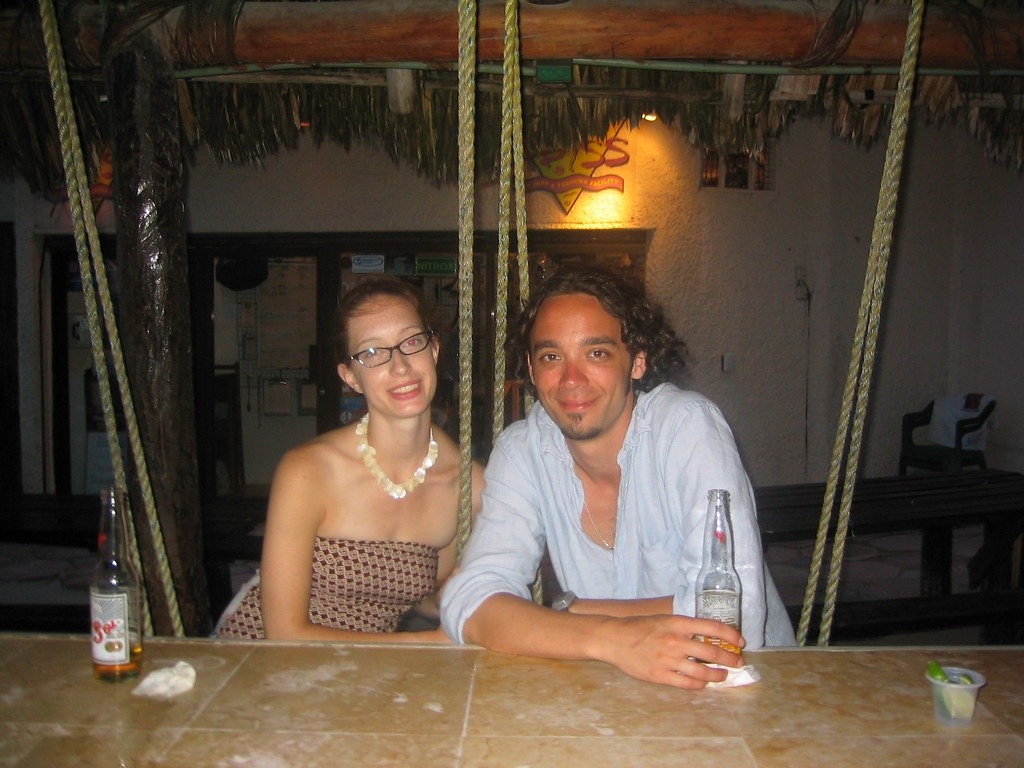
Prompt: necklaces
<box><xmin>356</xmin><ymin>410</ymin><xmax>438</xmax><ymax>500</ymax></box>
<box><xmin>585</xmin><ymin>501</ymin><xmax>617</xmax><ymax>550</ymax></box>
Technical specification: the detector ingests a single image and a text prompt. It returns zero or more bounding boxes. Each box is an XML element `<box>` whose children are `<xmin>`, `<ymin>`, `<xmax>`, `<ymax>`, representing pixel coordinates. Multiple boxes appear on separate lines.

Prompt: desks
<box><xmin>752</xmin><ymin>467</ymin><xmax>1024</xmax><ymax>645</ymax></box>
<box><xmin>0</xmin><ymin>633</ymin><xmax>1024</xmax><ymax>768</ymax></box>
<box><xmin>0</xmin><ymin>493</ymin><xmax>269</xmax><ymax>630</ymax></box>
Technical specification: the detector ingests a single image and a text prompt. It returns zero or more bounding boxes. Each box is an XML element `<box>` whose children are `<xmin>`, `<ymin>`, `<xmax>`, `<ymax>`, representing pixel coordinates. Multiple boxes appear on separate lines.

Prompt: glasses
<box><xmin>345</xmin><ymin>318</ymin><xmax>433</xmax><ymax>368</ymax></box>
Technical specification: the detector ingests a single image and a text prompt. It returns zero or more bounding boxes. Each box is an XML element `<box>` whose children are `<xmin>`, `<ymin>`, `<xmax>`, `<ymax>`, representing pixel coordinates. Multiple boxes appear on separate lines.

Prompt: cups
<box><xmin>926</xmin><ymin>667</ymin><xmax>986</xmax><ymax>726</ymax></box>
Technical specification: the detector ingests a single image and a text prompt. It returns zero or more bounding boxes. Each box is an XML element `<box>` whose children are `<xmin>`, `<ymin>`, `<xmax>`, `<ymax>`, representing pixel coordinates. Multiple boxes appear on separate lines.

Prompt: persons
<box><xmin>439</xmin><ymin>258</ymin><xmax>800</xmax><ymax>691</ymax></box>
<box><xmin>212</xmin><ymin>275</ymin><xmax>489</xmax><ymax>648</ymax></box>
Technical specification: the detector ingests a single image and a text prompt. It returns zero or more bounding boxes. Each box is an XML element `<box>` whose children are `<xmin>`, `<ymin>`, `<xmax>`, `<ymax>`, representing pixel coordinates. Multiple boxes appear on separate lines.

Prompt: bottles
<box><xmin>88</xmin><ymin>488</ymin><xmax>142</xmax><ymax>680</ymax></box>
<box><xmin>695</xmin><ymin>490</ymin><xmax>741</xmax><ymax>667</ymax></box>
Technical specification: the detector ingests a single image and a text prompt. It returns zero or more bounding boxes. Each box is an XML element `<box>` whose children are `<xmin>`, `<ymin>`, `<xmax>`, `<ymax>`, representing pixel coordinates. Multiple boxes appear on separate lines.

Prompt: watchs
<box><xmin>551</xmin><ymin>592</ymin><xmax>579</xmax><ymax>613</ymax></box>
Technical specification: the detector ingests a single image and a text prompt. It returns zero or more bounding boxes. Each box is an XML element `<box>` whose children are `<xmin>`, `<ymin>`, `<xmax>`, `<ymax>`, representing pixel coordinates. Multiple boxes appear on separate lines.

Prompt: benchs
<box><xmin>784</xmin><ymin>588</ymin><xmax>1024</xmax><ymax>645</ymax></box>
<box><xmin>0</xmin><ymin>604</ymin><xmax>91</xmax><ymax>633</ymax></box>
